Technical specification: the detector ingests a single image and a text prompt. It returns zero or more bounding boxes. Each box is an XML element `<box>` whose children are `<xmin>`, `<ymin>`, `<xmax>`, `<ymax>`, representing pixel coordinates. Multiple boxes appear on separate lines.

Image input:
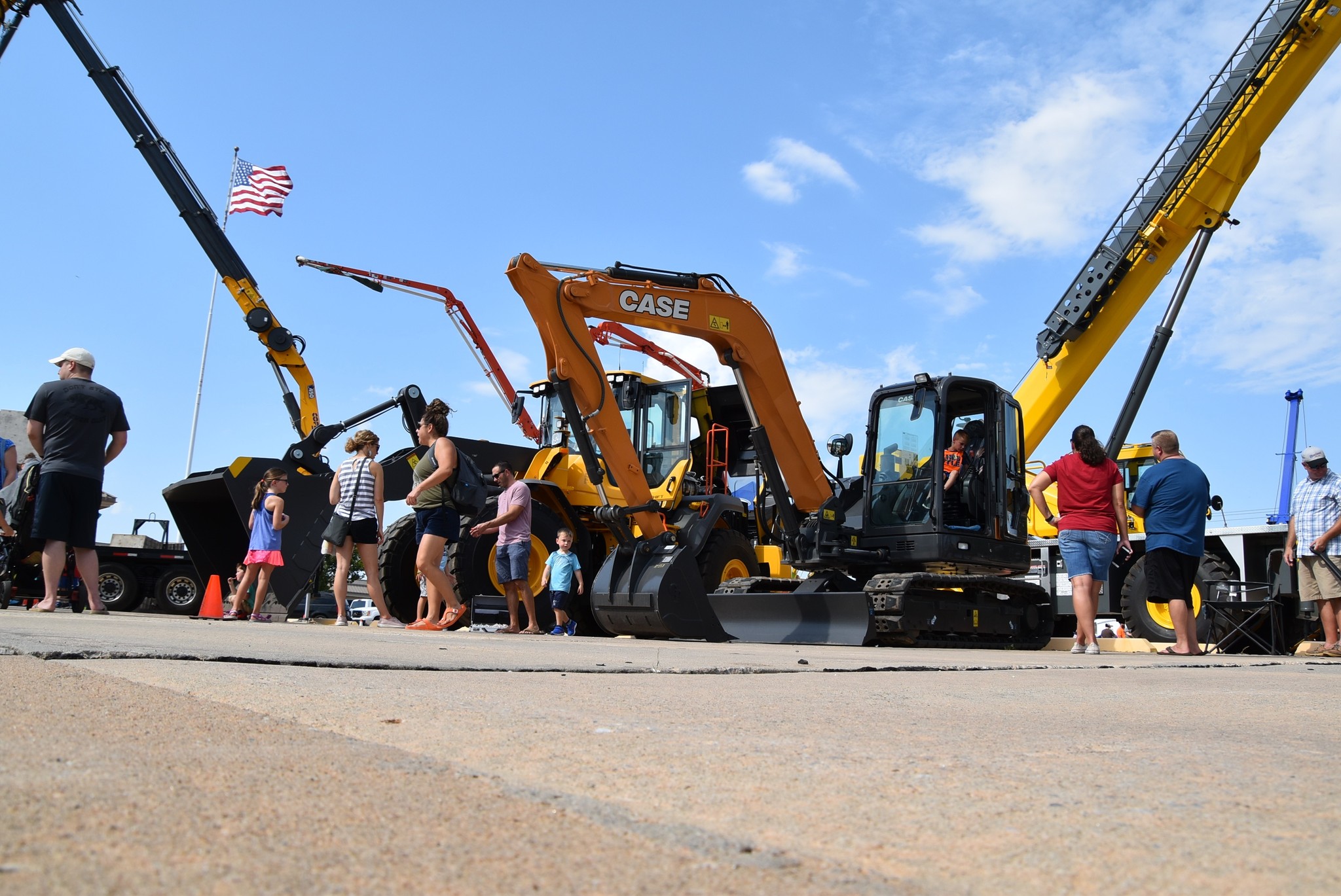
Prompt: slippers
<box><xmin>1156</xmin><ymin>646</ymin><xmax>1206</xmax><ymax>656</ymax></box>
<box><xmin>405</xmin><ymin>604</ymin><xmax>467</xmax><ymax>631</ymax></box>
<box><xmin>494</xmin><ymin>628</ymin><xmax>544</xmax><ymax>635</ymax></box>
<box><xmin>90</xmin><ymin>605</ymin><xmax>110</xmax><ymax>615</ymax></box>
<box><xmin>29</xmin><ymin>602</ymin><xmax>55</xmax><ymax>613</ymax></box>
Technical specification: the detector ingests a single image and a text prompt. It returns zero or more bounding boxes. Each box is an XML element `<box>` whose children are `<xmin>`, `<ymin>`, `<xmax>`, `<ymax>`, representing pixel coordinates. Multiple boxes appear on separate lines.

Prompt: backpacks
<box><xmin>429</xmin><ymin>439</ymin><xmax>486</xmax><ymax>518</ymax></box>
<box><xmin>10</xmin><ymin>463</ymin><xmax>40</xmax><ymax>522</ymax></box>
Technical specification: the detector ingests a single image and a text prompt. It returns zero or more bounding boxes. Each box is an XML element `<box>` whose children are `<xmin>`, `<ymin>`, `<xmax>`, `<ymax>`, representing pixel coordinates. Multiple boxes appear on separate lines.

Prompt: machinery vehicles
<box><xmin>1</xmin><ymin>1</ymin><xmax>1341</xmax><ymax>657</ymax></box>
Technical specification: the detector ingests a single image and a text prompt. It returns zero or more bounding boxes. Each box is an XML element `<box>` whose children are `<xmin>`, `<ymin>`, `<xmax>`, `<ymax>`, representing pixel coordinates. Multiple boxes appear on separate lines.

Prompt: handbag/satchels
<box><xmin>321</xmin><ymin>512</ymin><xmax>351</xmax><ymax>548</ymax></box>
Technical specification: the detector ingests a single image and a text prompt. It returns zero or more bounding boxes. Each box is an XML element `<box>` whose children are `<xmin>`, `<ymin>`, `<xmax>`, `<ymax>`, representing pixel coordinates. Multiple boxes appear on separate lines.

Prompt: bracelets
<box><xmin>1045</xmin><ymin>514</ymin><xmax>1054</xmax><ymax>522</ymax></box>
<box><xmin>1048</xmin><ymin>516</ymin><xmax>1055</xmax><ymax>524</ymax></box>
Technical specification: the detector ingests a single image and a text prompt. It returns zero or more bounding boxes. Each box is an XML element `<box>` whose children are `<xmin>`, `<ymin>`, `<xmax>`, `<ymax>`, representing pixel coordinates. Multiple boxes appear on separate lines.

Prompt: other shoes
<box><xmin>237</xmin><ymin>610</ymin><xmax>247</xmax><ymax>620</ymax></box>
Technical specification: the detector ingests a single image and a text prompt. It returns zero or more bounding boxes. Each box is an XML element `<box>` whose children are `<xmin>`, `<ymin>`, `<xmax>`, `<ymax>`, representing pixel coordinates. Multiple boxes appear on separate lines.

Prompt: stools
<box><xmin>642</xmin><ymin>451</ymin><xmax>665</xmax><ymax>486</ymax></box>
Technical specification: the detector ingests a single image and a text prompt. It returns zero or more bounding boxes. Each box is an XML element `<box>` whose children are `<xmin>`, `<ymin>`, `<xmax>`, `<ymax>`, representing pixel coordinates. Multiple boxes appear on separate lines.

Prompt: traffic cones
<box><xmin>189</xmin><ymin>575</ymin><xmax>226</xmax><ymax>619</ymax></box>
<box><xmin>360</xmin><ymin>619</ymin><xmax>363</xmax><ymax>626</ymax></box>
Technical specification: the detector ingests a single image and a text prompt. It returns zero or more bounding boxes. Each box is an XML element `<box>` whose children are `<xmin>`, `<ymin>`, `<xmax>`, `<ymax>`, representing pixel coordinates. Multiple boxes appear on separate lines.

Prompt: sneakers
<box><xmin>1071</xmin><ymin>642</ymin><xmax>1100</xmax><ymax>654</ymax></box>
<box><xmin>377</xmin><ymin>617</ymin><xmax>406</xmax><ymax>628</ymax></box>
<box><xmin>551</xmin><ymin>620</ymin><xmax>577</xmax><ymax>636</ymax></box>
<box><xmin>335</xmin><ymin>614</ymin><xmax>349</xmax><ymax>626</ymax></box>
<box><xmin>1305</xmin><ymin>643</ymin><xmax>1341</xmax><ymax>657</ymax></box>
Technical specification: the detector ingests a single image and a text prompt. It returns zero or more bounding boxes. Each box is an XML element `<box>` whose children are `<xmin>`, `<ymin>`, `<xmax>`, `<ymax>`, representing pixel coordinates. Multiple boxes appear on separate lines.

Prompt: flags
<box><xmin>227</xmin><ymin>157</ymin><xmax>294</xmax><ymax>218</ymax></box>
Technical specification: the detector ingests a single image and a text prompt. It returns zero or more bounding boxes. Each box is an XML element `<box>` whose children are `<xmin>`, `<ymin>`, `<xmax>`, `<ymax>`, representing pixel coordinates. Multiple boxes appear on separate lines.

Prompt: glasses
<box><xmin>492</xmin><ymin>471</ymin><xmax>503</xmax><ymax>478</ymax></box>
<box><xmin>276</xmin><ymin>479</ymin><xmax>287</xmax><ymax>484</ymax></box>
<box><xmin>1070</xmin><ymin>438</ymin><xmax>1074</xmax><ymax>443</ymax></box>
<box><xmin>418</xmin><ymin>422</ymin><xmax>429</xmax><ymax>430</ymax></box>
<box><xmin>372</xmin><ymin>443</ymin><xmax>380</xmax><ymax>451</ymax></box>
<box><xmin>1306</xmin><ymin>463</ymin><xmax>1327</xmax><ymax>470</ymax></box>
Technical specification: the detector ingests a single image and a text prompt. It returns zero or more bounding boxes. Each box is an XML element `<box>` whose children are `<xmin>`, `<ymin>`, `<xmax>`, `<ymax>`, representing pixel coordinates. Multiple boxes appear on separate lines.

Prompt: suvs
<box><xmin>349</xmin><ymin>598</ymin><xmax>381</xmax><ymax>621</ymax></box>
<box><xmin>291</xmin><ymin>591</ymin><xmax>352</xmax><ymax>621</ymax></box>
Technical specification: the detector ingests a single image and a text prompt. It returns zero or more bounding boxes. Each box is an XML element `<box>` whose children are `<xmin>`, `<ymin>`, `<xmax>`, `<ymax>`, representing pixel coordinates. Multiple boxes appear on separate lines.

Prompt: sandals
<box><xmin>249</xmin><ymin>613</ymin><xmax>272</xmax><ymax>623</ymax></box>
<box><xmin>223</xmin><ymin>610</ymin><xmax>238</xmax><ymax>620</ymax></box>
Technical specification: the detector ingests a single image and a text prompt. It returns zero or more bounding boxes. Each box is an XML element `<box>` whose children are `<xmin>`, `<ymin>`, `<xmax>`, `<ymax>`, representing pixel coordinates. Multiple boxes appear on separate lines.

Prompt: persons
<box><xmin>404</xmin><ymin>398</ymin><xmax>467</xmax><ymax>631</ymax></box>
<box><xmin>329</xmin><ymin>429</ymin><xmax>409</xmax><ymax>628</ymax></box>
<box><xmin>469</xmin><ymin>461</ymin><xmax>544</xmax><ymax>634</ymax></box>
<box><xmin>1283</xmin><ymin>446</ymin><xmax>1341</xmax><ymax>657</ymax></box>
<box><xmin>0</xmin><ymin>436</ymin><xmax>36</xmax><ymax>537</ymax></box>
<box><xmin>922</xmin><ymin>429</ymin><xmax>971</xmax><ymax>509</ymax></box>
<box><xmin>1028</xmin><ymin>425</ymin><xmax>1133</xmax><ymax>654</ymax></box>
<box><xmin>23</xmin><ymin>347</ymin><xmax>131</xmax><ymax>615</ymax></box>
<box><xmin>541</xmin><ymin>527</ymin><xmax>584</xmax><ymax>636</ymax></box>
<box><xmin>222</xmin><ymin>468</ymin><xmax>290</xmax><ymax>623</ymax></box>
<box><xmin>227</xmin><ymin>563</ymin><xmax>256</xmax><ymax>620</ymax></box>
<box><xmin>414</xmin><ymin>545</ymin><xmax>456</xmax><ymax>631</ymax></box>
<box><xmin>1131</xmin><ymin>429</ymin><xmax>1211</xmax><ymax>656</ymax></box>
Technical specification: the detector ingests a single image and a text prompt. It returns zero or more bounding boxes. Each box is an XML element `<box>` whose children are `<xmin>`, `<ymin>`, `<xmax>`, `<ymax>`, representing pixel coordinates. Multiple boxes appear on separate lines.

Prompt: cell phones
<box><xmin>1111</xmin><ymin>545</ymin><xmax>1131</xmax><ymax>568</ymax></box>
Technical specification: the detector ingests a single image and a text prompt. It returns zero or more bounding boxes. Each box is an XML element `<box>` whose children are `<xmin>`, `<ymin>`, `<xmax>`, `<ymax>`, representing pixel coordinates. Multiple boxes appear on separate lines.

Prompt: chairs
<box><xmin>917</xmin><ymin>418</ymin><xmax>985</xmax><ymax>523</ymax></box>
<box><xmin>1200</xmin><ymin>577</ymin><xmax>1285</xmax><ymax>656</ymax></box>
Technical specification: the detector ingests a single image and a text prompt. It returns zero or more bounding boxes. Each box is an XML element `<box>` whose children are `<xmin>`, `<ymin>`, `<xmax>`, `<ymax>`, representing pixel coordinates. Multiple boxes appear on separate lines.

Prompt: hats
<box><xmin>1105</xmin><ymin>623</ymin><xmax>1111</xmax><ymax>628</ymax></box>
<box><xmin>49</xmin><ymin>348</ymin><xmax>95</xmax><ymax>370</ymax></box>
<box><xmin>1301</xmin><ymin>446</ymin><xmax>1329</xmax><ymax>464</ymax></box>
